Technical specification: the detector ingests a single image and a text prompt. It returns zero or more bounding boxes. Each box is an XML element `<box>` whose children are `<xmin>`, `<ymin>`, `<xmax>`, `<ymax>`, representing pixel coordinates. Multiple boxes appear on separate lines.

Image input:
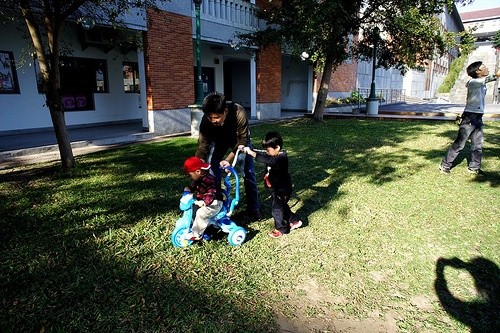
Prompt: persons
<box><xmin>238</xmin><ymin>132</ymin><xmax>303</xmax><ymax>238</ymax></box>
<box><xmin>439</xmin><ymin>61</ymin><xmax>500</xmax><ymax>176</ymax></box>
<box><xmin>195</xmin><ymin>93</ymin><xmax>264</xmax><ymax>220</ymax></box>
<box><xmin>181</xmin><ymin>157</ymin><xmax>223</xmax><ymax>240</ymax></box>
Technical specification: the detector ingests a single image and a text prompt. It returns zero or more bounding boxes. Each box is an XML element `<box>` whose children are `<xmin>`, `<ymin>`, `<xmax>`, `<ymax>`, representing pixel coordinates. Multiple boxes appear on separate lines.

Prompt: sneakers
<box><xmin>468</xmin><ymin>167</ymin><xmax>485</xmax><ymax>177</ymax></box>
<box><xmin>440</xmin><ymin>164</ymin><xmax>450</xmax><ymax>173</ymax></box>
<box><xmin>289</xmin><ymin>219</ymin><xmax>302</xmax><ymax>231</ymax></box>
<box><xmin>244</xmin><ymin>208</ymin><xmax>267</xmax><ymax>221</ymax></box>
<box><xmin>269</xmin><ymin>228</ymin><xmax>283</xmax><ymax>238</ymax></box>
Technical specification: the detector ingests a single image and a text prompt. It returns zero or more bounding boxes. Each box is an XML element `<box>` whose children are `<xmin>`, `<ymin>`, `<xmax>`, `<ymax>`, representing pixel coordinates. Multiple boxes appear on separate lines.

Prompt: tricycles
<box><xmin>171</xmin><ymin>145</ymin><xmax>249</xmax><ymax>249</ymax></box>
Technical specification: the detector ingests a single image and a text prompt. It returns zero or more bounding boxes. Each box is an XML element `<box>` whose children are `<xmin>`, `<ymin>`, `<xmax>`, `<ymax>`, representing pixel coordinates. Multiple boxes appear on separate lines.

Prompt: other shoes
<box><xmin>183</xmin><ymin>232</ymin><xmax>202</xmax><ymax>241</ymax></box>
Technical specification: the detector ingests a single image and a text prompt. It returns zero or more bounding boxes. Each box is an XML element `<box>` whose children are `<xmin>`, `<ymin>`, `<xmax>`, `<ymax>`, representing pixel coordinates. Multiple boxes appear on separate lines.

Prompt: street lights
<box><xmin>368</xmin><ymin>25</ymin><xmax>381</xmax><ymax>98</ymax></box>
<box><xmin>193</xmin><ymin>0</ymin><xmax>205</xmax><ymax>105</ymax></box>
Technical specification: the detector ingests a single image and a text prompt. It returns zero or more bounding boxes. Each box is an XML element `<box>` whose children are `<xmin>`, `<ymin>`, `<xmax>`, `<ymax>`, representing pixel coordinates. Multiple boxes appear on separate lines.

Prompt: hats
<box><xmin>184</xmin><ymin>155</ymin><xmax>211</xmax><ymax>173</ymax></box>
